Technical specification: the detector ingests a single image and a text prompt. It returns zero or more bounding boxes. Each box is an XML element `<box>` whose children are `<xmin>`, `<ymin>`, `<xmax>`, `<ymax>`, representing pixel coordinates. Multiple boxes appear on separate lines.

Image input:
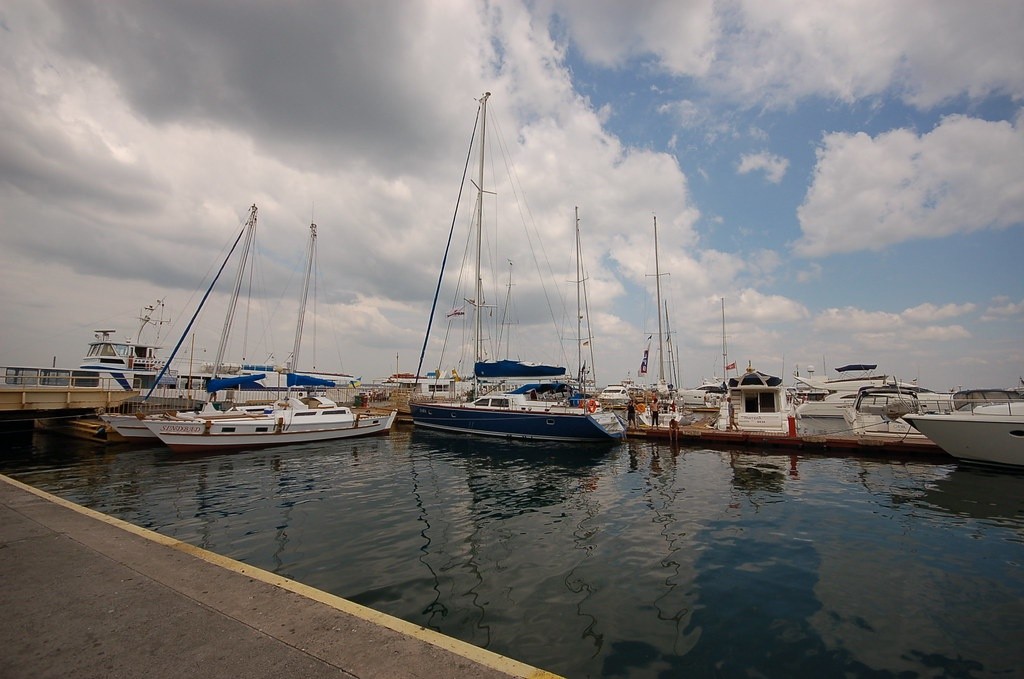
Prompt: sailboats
<box><xmin>409</xmin><ymin>87</ymin><xmax>628</xmax><ymax>441</ymax></box>
<box><xmin>99</xmin><ymin>197</ymin><xmax>401</xmax><ymax>447</ymax></box>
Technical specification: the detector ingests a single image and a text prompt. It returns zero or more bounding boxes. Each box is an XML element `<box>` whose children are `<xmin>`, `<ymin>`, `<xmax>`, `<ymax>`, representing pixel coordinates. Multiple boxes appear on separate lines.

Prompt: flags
<box><xmin>726</xmin><ymin>362</ymin><xmax>736</xmax><ymax>370</ymax></box>
<box><xmin>348</xmin><ymin>377</ymin><xmax>362</xmax><ymax>390</ymax></box>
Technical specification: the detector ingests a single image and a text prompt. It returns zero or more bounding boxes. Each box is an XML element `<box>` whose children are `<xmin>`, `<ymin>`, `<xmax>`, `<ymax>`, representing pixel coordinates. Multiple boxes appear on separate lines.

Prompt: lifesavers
<box><xmin>588</xmin><ymin>399</ymin><xmax>597</xmax><ymax>413</ymax></box>
<box><xmin>637</xmin><ymin>404</ymin><xmax>645</xmax><ymax>412</ymax></box>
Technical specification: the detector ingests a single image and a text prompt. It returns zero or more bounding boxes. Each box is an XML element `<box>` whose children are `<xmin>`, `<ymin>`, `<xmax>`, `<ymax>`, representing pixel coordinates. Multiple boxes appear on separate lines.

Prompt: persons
<box><xmin>209</xmin><ymin>391</ymin><xmax>217</xmax><ymax>402</ymax></box>
<box><xmin>649</xmin><ymin>398</ymin><xmax>660</xmax><ymax>430</ymax></box>
<box><xmin>624</xmin><ymin>399</ymin><xmax>642</xmax><ymax>429</ymax></box>
<box><xmin>725</xmin><ymin>397</ymin><xmax>739</xmax><ymax>432</ymax></box>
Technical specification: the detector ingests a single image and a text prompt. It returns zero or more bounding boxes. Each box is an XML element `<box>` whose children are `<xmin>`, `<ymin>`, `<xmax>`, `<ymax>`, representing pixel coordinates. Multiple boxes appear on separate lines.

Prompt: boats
<box><xmin>597</xmin><ymin>293</ymin><xmax>917</xmax><ymax>437</ymax></box>
<box><xmin>901</xmin><ymin>393</ymin><xmax>1024</xmax><ymax>468</ymax></box>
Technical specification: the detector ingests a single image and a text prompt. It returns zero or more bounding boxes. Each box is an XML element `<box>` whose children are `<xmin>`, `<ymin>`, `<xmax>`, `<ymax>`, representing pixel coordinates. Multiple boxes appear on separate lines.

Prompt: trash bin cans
<box><xmin>354</xmin><ymin>392</ymin><xmax>368</xmax><ymax>407</ymax></box>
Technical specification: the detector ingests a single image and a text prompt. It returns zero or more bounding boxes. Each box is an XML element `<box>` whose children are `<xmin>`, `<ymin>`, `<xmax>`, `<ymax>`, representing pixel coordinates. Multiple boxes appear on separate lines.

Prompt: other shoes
<box><xmin>649</xmin><ymin>428</ymin><xmax>653</xmax><ymax>430</ymax></box>
<box><xmin>725</xmin><ymin>430</ymin><xmax>732</xmax><ymax>432</ymax></box>
<box><xmin>736</xmin><ymin>428</ymin><xmax>738</xmax><ymax>432</ymax></box>
<box><xmin>657</xmin><ymin>428</ymin><xmax>659</xmax><ymax>430</ymax></box>
